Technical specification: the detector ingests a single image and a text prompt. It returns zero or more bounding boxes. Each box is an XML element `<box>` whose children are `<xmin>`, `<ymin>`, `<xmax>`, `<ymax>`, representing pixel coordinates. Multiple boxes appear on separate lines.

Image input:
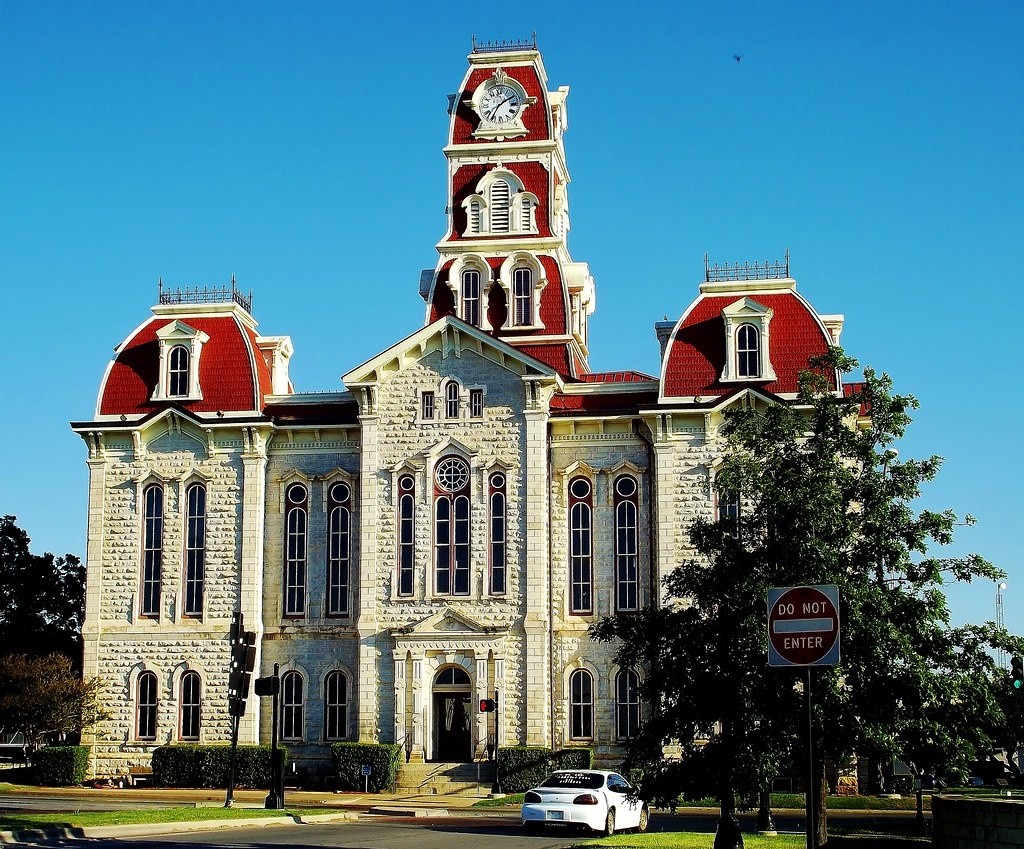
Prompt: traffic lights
<box><xmin>481</xmin><ymin>699</ymin><xmax>494</xmax><ymax>712</ymax></box>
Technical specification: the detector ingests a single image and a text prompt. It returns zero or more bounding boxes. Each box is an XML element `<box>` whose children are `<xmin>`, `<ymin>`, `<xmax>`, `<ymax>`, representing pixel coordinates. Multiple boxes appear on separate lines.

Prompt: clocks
<box><xmin>478</xmin><ymin>82</ymin><xmax>522</xmax><ymax>125</ymax></box>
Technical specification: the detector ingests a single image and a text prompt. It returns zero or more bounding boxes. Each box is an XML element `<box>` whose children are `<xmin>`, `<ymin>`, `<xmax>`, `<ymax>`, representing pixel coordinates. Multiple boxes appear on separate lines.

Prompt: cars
<box><xmin>521</xmin><ymin>769</ymin><xmax>650</xmax><ymax>837</ymax></box>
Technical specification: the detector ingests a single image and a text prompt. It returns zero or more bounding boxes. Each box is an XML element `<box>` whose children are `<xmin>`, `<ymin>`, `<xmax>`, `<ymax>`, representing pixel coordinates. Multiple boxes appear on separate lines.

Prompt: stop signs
<box><xmin>768</xmin><ymin>586</ymin><xmax>840</xmax><ymax>666</ymax></box>
